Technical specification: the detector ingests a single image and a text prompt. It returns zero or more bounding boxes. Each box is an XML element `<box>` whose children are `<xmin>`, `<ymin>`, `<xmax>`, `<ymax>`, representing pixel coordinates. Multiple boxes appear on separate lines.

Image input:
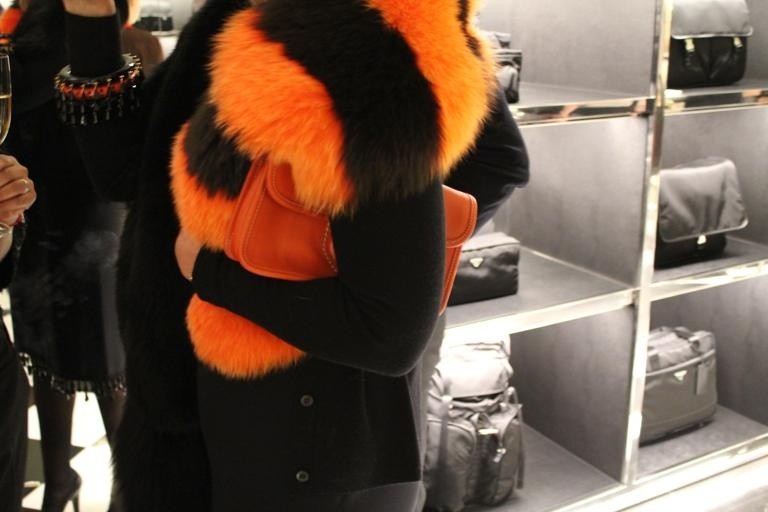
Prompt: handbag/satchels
<box><xmin>639</xmin><ymin>325</ymin><xmax>718</xmax><ymax>444</ymax></box>
<box><xmin>224</xmin><ymin>145</ymin><xmax>478</xmax><ymax>316</ymax></box>
<box><xmin>667</xmin><ymin>0</ymin><xmax>754</xmax><ymax>89</ymax></box>
<box><xmin>447</xmin><ymin>231</ymin><xmax>520</xmax><ymax>307</ymax></box>
<box><xmin>477</xmin><ymin>30</ymin><xmax>522</xmax><ymax>103</ymax></box>
<box><xmin>654</xmin><ymin>158</ymin><xmax>749</xmax><ymax>269</ymax></box>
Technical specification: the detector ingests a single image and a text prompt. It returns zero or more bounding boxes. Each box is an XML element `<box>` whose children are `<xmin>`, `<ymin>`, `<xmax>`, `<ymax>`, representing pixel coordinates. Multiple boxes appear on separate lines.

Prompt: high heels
<box><xmin>41</xmin><ymin>466</ymin><xmax>82</xmax><ymax>512</ymax></box>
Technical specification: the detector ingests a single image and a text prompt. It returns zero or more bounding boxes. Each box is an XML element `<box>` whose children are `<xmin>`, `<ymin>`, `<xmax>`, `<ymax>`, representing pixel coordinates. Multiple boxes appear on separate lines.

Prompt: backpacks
<box><xmin>420</xmin><ymin>335</ymin><xmax>523</xmax><ymax>512</ymax></box>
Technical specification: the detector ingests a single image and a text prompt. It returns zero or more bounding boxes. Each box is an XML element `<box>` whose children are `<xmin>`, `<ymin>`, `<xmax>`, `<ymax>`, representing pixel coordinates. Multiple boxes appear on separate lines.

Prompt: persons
<box><xmin>49</xmin><ymin>0</ymin><xmax>504</xmax><ymax>512</ymax></box>
<box><xmin>345</xmin><ymin>70</ymin><xmax>532</xmax><ymax>512</ymax></box>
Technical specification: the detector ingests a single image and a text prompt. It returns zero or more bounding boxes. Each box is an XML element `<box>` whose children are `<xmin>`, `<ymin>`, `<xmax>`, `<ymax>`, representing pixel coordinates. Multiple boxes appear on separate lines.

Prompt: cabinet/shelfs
<box><xmin>418</xmin><ymin>0</ymin><xmax>768</xmax><ymax>512</ymax></box>
<box><xmin>127</xmin><ymin>2</ymin><xmax>207</xmax><ymax>62</ymax></box>
